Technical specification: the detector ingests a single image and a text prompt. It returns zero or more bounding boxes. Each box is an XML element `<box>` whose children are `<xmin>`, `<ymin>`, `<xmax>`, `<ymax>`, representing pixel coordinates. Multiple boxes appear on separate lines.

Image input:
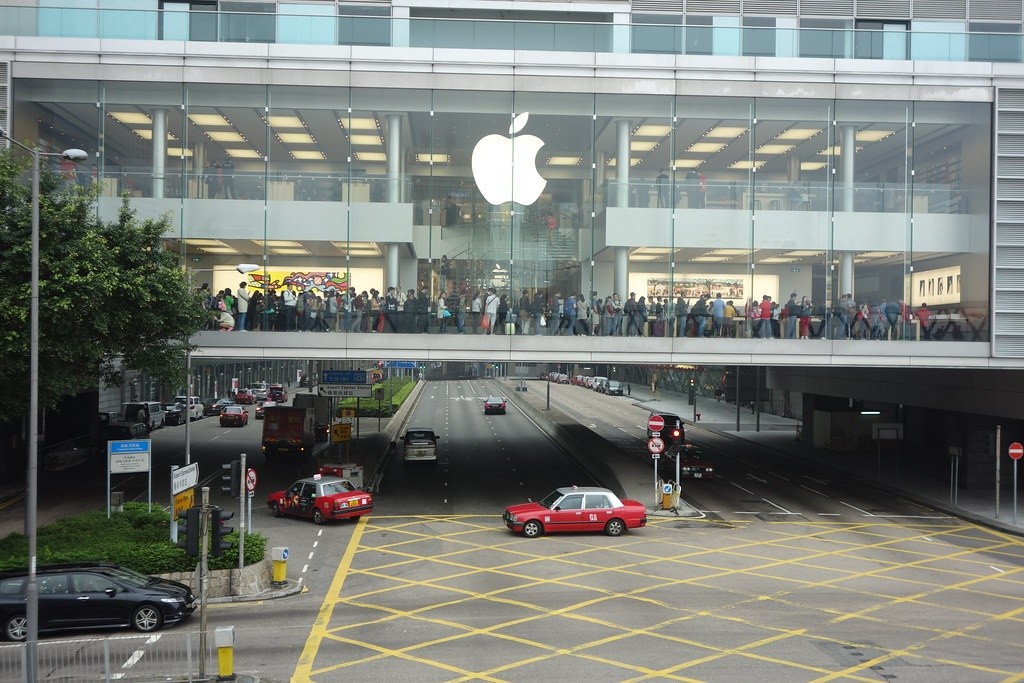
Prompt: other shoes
<box><xmin>220</xmin><ymin>329</ymin><xmax>226</xmax><ymax>331</ymax></box>
<box><xmin>762</xmin><ymin>336</ymin><xmax>881</xmax><ymax>341</ymax></box>
<box><xmin>227</xmin><ymin>327</ymin><xmax>233</xmax><ymax>331</ymax></box>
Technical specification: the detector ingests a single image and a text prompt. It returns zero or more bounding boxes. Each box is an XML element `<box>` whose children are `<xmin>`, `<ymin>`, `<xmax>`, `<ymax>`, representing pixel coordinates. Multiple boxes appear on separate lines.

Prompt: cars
<box><xmin>502</xmin><ymin>485</ymin><xmax>647</xmax><ymax>538</ymax></box>
<box><xmin>165</xmin><ymin>382</ymin><xmax>289</xmax><ymax>427</ymax></box>
<box><xmin>539</xmin><ymin>372</ymin><xmax>625</xmax><ymax>396</ymax></box>
<box><xmin>96</xmin><ymin>412</ymin><xmax>125</xmax><ymax>425</ymax></box>
<box><xmin>483</xmin><ymin>397</ymin><xmax>507</xmax><ymax>415</ymax></box>
<box><xmin>266</xmin><ymin>474</ymin><xmax>374</xmax><ymax>525</ymax></box>
<box><xmin>663</xmin><ymin>444</ymin><xmax>715</xmax><ymax>482</ymax></box>
<box><xmin>300</xmin><ymin>373</ymin><xmax>319</xmax><ymax>388</ymax></box>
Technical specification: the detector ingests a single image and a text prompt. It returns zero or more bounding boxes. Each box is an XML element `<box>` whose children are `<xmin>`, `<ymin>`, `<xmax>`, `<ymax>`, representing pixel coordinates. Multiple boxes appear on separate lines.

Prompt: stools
<box><xmin>802</xmin><ymin>201</ymin><xmax>810</xmax><ymax>210</ymax></box>
<box><xmin>752</xmin><ymin>199</ymin><xmax>761</xmax><ymax>210</ymax></box>
<box><xmin>770</xmin><ymin>199</ymin><xmax>780</xmax><ymax>210</ymax></box>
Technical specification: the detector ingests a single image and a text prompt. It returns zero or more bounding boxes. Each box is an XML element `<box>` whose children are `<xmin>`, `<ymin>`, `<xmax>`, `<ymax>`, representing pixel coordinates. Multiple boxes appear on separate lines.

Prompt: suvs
<box><xmin>0</xmin><ymin>563</ymin><xmax>198</xmax><ymax>643</ymax></box>
<box><xmin>647</xmin><ymin>411</ymin><xmax>686</xmax><ymax>444</ymax></box>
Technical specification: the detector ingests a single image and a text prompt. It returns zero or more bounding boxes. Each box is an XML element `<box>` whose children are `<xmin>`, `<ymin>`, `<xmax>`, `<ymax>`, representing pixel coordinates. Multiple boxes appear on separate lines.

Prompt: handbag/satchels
<box><xmin>443</xmin><ymin>310</ymin><xmax>451</xmax><ymax>318</ymax></box>
<box><xmin>540</xmin><ymin>317</ymin><xmax>546</xmax><ymax>326</ymax></box>
<box><xmin>481</xmin><ymin>315</ymin><xmax>488</xmax><ymax>329</ymax></box>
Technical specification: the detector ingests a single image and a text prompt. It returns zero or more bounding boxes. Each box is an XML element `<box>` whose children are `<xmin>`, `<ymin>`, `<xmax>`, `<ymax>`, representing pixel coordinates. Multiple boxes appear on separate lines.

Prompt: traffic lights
<box><xmin>689</xmin><ymin>378</ymin><xmax>694</xmax><ymax>388</ymax></box>
<box><xmin>672</xmin><ymin>429</ymin><xmax>681</xmax><ymax>453</ymax></box>
<box><xmin>176</xmin><ymin>508</ymin><xmax>201</xmax><ymax>557</ymax></box>
<box><xmin>212</xmin><ymin>508</ymin><xmax>234</xmax><ymax>556</ymax></box>
<box><xmin>221</xmin><ymin>459</ymin><xmax>241</xmax><ymax>498</ymax></box>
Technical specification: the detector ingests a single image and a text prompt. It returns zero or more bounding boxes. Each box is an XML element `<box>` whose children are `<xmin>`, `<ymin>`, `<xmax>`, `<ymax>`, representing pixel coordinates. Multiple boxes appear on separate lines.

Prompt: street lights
<box><xmin>24</xmin><ymin>147</ymin><xmax>90</xmax><ymax>683</ymax></box>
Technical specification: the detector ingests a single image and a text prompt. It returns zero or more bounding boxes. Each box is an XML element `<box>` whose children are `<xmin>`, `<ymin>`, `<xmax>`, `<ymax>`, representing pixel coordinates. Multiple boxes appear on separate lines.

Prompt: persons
<box><xmin>715</xmin><ymin>386</ymin><xmax>722</xmax><ymax>402</ymax></box>
<box><xmin>203</xmin><ymin>157</ymin><xmax>237</xmax><ymax>199</ymax></box>
<box><xmin>227</xmin><ymin>388</ymin><xmax>236</xmax><ymax>399</ymax></box>
<box><xmin>547</xmin><ymin>212</ymin><xmax>556</xmax><ymax>246</ymax></box>
<box><xmin>197</xmin><ymin>281</ymin><xmax>383</xmax><ymax>332</ymax></box>
<box><xmin>440</xmin><ymin>254</ymin><xmax>448</xmax><ymax>292</ymax></box>
<box><xmin>685</xmin><ymin>167</ymin><xmax>706</xmax><ymax>208</ymax></box>
<box><xmin>384</xmin><ymin>285</ymin><xmax>449</xmax><ymax>333</ymax></box>
<box><xmin>628</xmin><ymin>168</ymin><xmax>669</xmax><ymax>208</ymax></box>
<box><xmin>628</xmin><ymin>382</ymin><xmax>632</xmax><ymax>395</ymax></box>
<box><xmin>590</xmin><ymin>291</ymin><xmax>737</xmax><ymax>338</ymax></box>
<box><xmin>833</xmin><ymin>293</ymin><xmax>931</xmax><ymax>338</ymax></box>
<box><xmin>60</xmin><ymin>157</ymin><xmax>98</xmax><ymax>188</ymax></box>
<box><xmin>370</xmin><ymin>180</ymin><xmax>382</xmax><ymax>202</ymax></box>
<box><xmin>786</xmin><ymin>179</ymin><xmax>804</xmax><ymax>209</ymax></box>
<box><xmin>125</xmin><ymin>173</ymin><xmax>135</xmax><ymax>195</ymax></box>
<box><xmin>744</xmin><ymin>292</ymin><xmax>816</xmax><ymax>339</ymax></box>
<box><xmin>457</xmin><ymin>287</ymin><xmax>588</xmax><ymax>336</ymax></box>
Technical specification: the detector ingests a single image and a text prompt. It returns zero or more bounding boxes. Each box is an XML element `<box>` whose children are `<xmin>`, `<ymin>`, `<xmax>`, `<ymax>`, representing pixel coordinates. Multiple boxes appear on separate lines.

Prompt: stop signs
<box><xmin>1008</xmin><ymin>442</ymin><xmax>1023</xmax><ymax>460</ymax></box>
<box><xmin>648</xmin><ymin>415</ymin><xmax>664</xmax><ymax>432</ymax></box>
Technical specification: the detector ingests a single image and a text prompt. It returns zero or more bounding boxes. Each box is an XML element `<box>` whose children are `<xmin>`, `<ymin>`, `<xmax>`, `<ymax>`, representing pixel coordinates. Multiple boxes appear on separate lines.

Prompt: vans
<box><xmin>399</xmin><ymin>427</ymin><xmax>440</xmax><ymax>466</ymax></box>
<box><xmin>96</xmin><ymin>421</ymin><xmax>151</xmax><ymax>458</ymax></box>
<box><xmin>122</xmin><ymin>401</ymin><xmax>166</xmax><ymax>431</ymax></box>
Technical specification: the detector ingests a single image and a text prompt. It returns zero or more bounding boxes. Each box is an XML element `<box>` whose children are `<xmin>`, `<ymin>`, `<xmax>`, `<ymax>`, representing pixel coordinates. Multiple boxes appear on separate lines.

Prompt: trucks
<box><xmin>293</xmin><ymin>393</ymin><xmax>330</xmax><ymax>443</ymax></box>
<box><xmin>261</xmin><ymin>406</ymin><xmax>319</xmax><ymax>465</ymax></box>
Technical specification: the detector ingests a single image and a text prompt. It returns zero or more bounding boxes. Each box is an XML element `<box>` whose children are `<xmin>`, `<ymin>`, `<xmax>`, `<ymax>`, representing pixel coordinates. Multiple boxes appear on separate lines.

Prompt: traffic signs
<box><xmin>332</xmin><ymin>423</ymin><xmax>351</xmax><ymax>442</ymax></box>
<box><xmin>322</xmin><ymin>370</ymin><xmax>367</xmax><ymax>383</ymax></box>
<box><xmin>317</xmin><ymin>384</ymin><xmax>372</xmax><ymax>397</ymax></box>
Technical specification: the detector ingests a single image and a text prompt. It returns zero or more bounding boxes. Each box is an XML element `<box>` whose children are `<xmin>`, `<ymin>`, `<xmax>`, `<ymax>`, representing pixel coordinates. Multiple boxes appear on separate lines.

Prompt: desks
<box><xmin>643</xmin><ymin>316</ymin><xmax>964</xmax><ymax>337</ymax></box>
<box><xmin>648</xmin><ymin>191</ymin><xmax>928</xmax><ymax>210</ymax></box>
<box><xmin>96</xmin><ymin>177</ymin><xmax>371</xmax><ymax>202</ymax></box>
